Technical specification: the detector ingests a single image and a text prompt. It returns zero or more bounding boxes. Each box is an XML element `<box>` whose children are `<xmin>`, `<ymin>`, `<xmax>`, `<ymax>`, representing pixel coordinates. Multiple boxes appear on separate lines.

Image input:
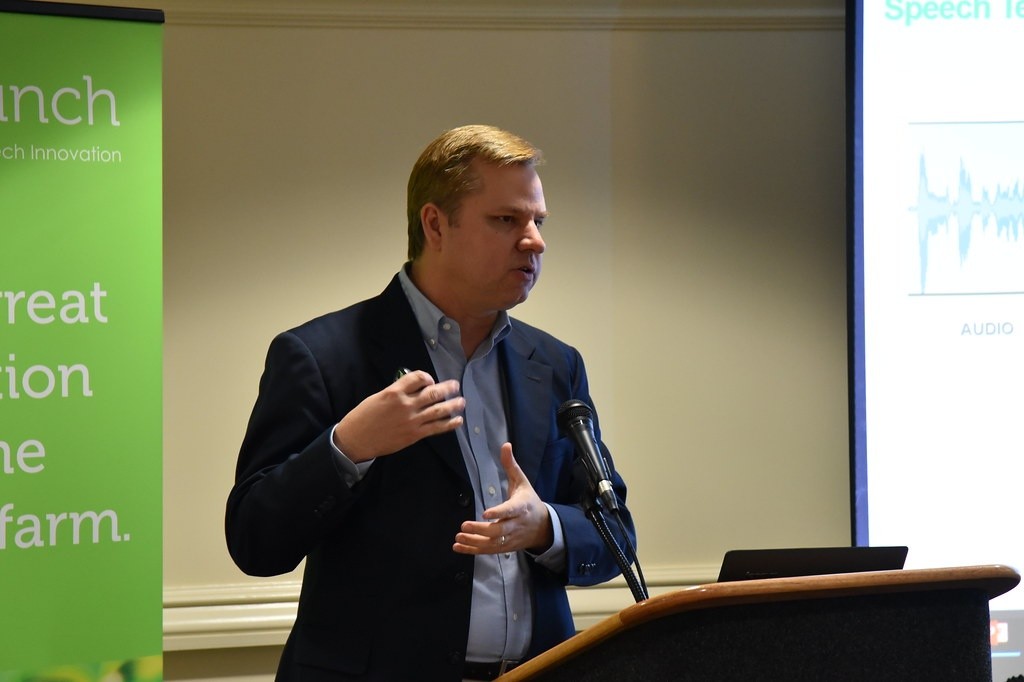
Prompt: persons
<box><xmin>225</xmin><ymin>125</ymin><xmax>637</xmax><ymax>682</ymax></box>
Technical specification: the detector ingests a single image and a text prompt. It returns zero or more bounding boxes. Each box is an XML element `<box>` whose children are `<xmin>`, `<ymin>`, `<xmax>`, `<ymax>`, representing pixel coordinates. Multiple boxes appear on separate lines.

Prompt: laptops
<box><xmin>715</xmin><ymin>546</ymin><xmax>908</xmax><ymax>584</ymax></box>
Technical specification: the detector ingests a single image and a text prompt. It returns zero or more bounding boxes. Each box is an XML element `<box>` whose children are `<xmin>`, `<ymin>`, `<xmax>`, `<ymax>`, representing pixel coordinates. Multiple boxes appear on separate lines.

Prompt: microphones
<box><xmin>556</xmin><ymin>400</ymin><xmax>619</xmax><ymax>515</ymax></box>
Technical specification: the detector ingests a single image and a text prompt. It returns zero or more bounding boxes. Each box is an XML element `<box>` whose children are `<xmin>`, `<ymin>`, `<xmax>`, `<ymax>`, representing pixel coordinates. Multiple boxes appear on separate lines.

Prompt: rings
<box><xmin>500</xmin><ymin>537</ymin><xmax>505</xmax><ymax>547</ymax></box>
